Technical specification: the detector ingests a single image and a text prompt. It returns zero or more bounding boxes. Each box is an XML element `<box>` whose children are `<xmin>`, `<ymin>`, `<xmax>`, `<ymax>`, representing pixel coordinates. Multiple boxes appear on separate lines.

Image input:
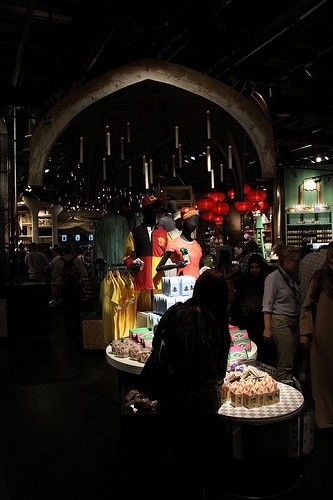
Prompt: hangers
<box><xmin>104</xmin><ymin>263</ymin><xmax>129</xmax><ymax>280</ymax></box>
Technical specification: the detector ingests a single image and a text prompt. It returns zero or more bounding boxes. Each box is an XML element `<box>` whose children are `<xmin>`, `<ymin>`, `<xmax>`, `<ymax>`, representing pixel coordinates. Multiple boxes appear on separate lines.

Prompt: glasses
<box><xmin>288</xmin><ymin>258</ymin><xmax>301</xmax><ymax>263</ymax></box>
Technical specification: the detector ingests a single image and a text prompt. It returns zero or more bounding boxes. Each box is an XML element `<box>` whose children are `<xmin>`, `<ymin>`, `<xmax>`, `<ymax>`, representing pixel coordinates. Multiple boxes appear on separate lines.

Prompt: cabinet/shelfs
<box><xmin>285</xmin><ymin>212</ymin><xmax>333</xmax><ymax>249</ymax></box>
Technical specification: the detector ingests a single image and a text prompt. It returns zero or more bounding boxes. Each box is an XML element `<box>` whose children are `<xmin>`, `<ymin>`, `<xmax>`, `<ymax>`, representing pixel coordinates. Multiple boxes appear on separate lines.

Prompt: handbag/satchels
<box><xmin>243</xmin><ymin>307</ymin><xmax>263</xmax><ymax>333</ymax></box>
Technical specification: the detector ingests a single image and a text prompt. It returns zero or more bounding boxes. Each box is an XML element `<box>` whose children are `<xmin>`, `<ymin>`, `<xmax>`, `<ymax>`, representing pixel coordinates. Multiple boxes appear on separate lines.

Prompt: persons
<box><xmin>261</xmin><ymin>241</ymin><xmax>305</xmax><ymax>379</ymax></box>
<box><xmin>238</xmin><ymin>254</ymin><xmax>273</xmax><ymax>361</ymax></box>
<box><xmin>157</xmin><ymin>213</ymin><xmax>202</xmax><ymax>276</ymax></box>
<box><xmin>18</xmin><ymin>243</ymin><xmax>94</xmax><ymax>305</ymax></box>
<box><xmin>94</xmin><ymin>199</ymin><xmax>182</xmax><ymax>314</ymax></box>
<box><xmin>139</xmin><ymin>268</ymin><xmax>231</xmax><ymax>500</ymax></box>
<box><xmin>299</xmin><ymin>245</ymin><xmax>329</xmax><ymax>347</ymax></box>
<box><xmin>51</xmin><ymin>244</ymin><xmax>88</xmax><ymax>318</ymax></box>
<box><xmin>300</xmin><ymin>241</ymin><xmax>333</xmax><ymax>428</ymax></box>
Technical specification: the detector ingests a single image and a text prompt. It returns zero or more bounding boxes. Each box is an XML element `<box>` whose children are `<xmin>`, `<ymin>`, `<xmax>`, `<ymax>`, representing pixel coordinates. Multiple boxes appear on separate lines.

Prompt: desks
<box><xmin>105</xmin><ymin>331</ymin><xmax>305</xmax><ymax>500</ymax></box>
